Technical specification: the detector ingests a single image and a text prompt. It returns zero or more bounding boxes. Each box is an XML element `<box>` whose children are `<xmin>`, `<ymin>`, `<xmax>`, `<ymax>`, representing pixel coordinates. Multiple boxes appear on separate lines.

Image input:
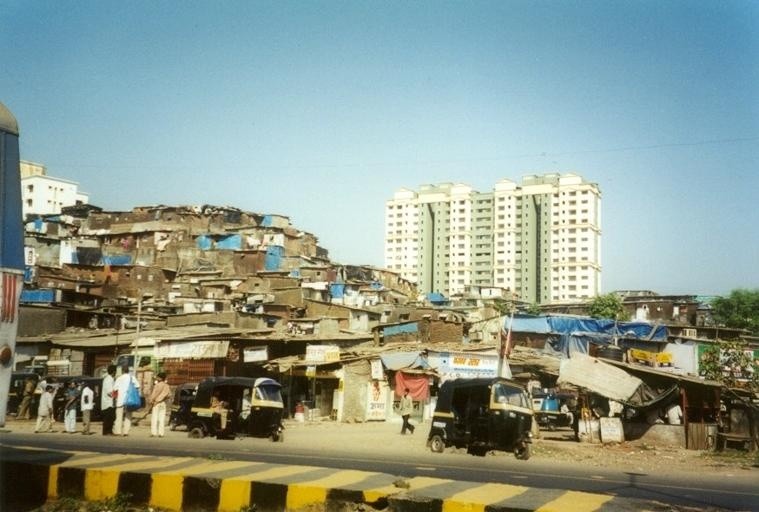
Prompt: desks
<box><xmin>717</xmin><ymin>432</ymin><xmax>752</xmax><ymax>450</ymax></box>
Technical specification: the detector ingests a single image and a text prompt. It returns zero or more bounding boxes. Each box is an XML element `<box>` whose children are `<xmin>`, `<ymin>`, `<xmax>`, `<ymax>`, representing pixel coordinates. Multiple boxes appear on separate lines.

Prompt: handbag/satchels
<box><xmin>123</xmin><ymin>375</ymin><xmax>142</xmax><ymax>410</ymax></box>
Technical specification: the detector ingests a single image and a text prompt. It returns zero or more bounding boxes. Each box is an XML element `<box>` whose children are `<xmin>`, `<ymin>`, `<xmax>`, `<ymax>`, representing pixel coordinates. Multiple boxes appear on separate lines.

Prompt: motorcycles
<box><xmin>426</xmin><ymin>376</ymin><xmax>533</xmax><ymax>461</ymax></box>
<box><xmin>8</xmin><ymin>373</ymin><xmax>146</xmax><ymax>422</ymax></box>
<box><xmin>531</xmin><ymin>392</ymin><xmax>577</xmax><ymax>431</ymax></box>
<box><xmin>185</xmin><ymin>376</ymin><xmax>285</xmax><ymax>442</ymax></box>
<box><xmin>168</xmin><ymin>383</ymin><xmax>201</xmax><ymax>432</ymax></box>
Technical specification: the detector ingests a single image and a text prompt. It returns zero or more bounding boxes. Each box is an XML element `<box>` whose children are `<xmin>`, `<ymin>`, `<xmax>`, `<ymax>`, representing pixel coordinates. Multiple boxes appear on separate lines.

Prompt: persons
<box><xmin>645</xmin><ymin>406</ymin><xmax>664</xmax><ymax>424</ymax></box>
<box><xmin>399</xmin><ymin>387</ymin><xmax>415</xmax><ymax>435</ymax></box>
<box><xmin>664</xmin><ymin>401</ymin><xmax>683</xmax><ymax>425</ymax></box>
<box><xmin>15</xmin><ymin>377</ymin><xmax>95</xmax><ymax>435</ymax></box>
<box><xmin>149</xmin><ymin>372</ymin><xmax>171</xmax><ymax>437</ymax></box>
<box><xmin>210</xmin><ymin>389</ymin><xmax>228</xmax><ymax>430</ymax></box>
<box><xmin>100</xmin><ymin>366</ymin><xmax>117</xmax><ymax>436</ymax></box>
<box><xmin>112</xmin><ymin>364</ymin><xmax>140</xmax><ymax>437</ymax></box>
<box><xmin>560</xmin><ymin>399</ymin><xmax>574</xmax><ymax>425</ymax></box>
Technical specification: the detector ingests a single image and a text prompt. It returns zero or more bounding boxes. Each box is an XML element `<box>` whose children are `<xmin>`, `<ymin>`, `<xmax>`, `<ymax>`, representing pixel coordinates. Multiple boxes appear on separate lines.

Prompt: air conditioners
<box><xmin>682</xmin><ymin>328</ymin><xmax>697</xmax><ymax>338</ymax></box>
